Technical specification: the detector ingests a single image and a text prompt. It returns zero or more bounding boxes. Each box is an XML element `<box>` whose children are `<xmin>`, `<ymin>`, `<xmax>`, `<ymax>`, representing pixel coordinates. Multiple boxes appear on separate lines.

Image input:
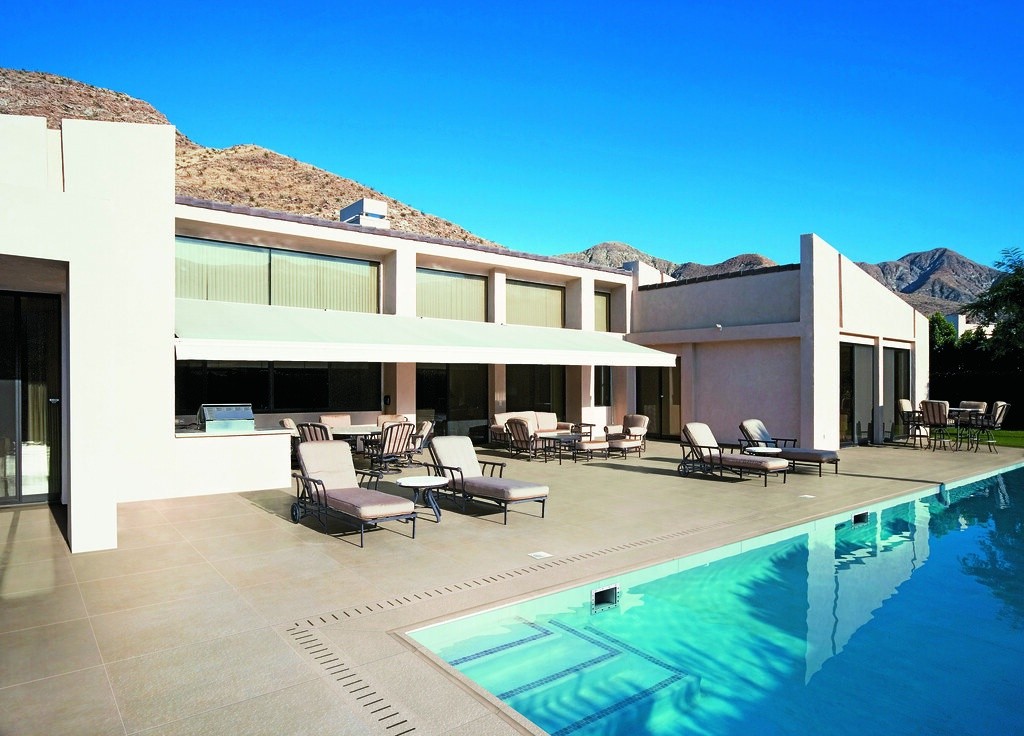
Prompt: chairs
<box><xmin>678</xmin><ymin>418</ymin><xmax>840</xmax><ymax>487</ymax></box>
<box><xmin>428</xmin><ymin>435</ymin><xmax>549</xmax><ymax>525</ymax></box>
<box><xmin>504</xmin><ymin>417</ymin><xmax>538</xmax><ymax>462</ymax></box>
<box><xmin>604</xmin><ymin>415</ymin><xmax>650</xmax><ymax>452</ymax></box>
<box><xmin>898</xmin><ymin>399</ymin><xmax>1010</xmax><ymax>454</ymax></box>
<box><xmin>279</xmin><ymin>414</ymin><xmax>436</xmax><ymax>475</ymax></box>
<box><xmin>291</xmin><ymin>440</ymin><xmax>417</xmax><ymax>548</ymax></box>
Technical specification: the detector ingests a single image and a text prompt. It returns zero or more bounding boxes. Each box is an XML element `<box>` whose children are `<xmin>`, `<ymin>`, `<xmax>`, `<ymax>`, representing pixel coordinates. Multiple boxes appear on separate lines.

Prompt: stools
<box><xmin>574</xmin><ymin>441</ymin><xmax>609</xmax><ymax>463</ymax></box>
<box><xmin>609</xmin><ymin>440</ymin><xmax>641</xmax><ymax>460</ymax></box>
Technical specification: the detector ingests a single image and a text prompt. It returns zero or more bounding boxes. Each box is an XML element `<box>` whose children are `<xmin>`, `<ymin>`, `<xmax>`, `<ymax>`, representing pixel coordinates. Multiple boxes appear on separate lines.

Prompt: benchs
<box><xmin>489</xmin><ymin>411</ymin><xmax>575</xmax><ymax>452</ymax></box>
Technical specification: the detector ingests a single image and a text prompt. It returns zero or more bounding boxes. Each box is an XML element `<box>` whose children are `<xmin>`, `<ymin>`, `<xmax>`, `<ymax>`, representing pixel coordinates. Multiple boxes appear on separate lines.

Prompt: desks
<box><xmin>947</xmin><ymin>408</ymin><xmax>984</xmax><ymax>451</ymax></box>
<box><xmin>539</xmin><ymin>435</ymin><xmax>582</xmax><ymax>465</ymax></box>
<box><xmin>329</xmin><ymin>425</ymin><xmax>381</xmax><ymax>456</ymax></box>
<box><xmin>746</xmin><ymin>447</ymin><xmax>782</xmax><ymax>458</ymax></box>
<box><xmin>396</xmin><ymin>476</ymin><xmax>449</xmax><ymax>523</ymax></box>
